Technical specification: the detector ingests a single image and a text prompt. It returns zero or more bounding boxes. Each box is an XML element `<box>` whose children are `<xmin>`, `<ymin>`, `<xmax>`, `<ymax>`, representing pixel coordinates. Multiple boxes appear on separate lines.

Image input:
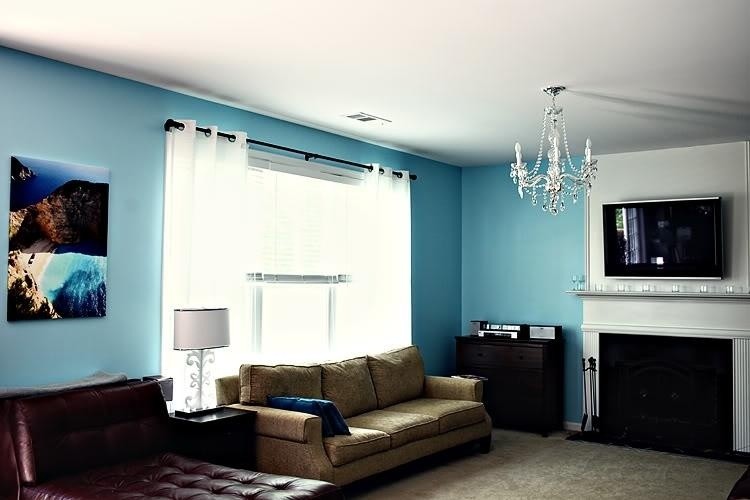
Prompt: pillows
<box><xmin>267</xmin><ymin>395</ymin><xmax>352</xmax><ymax>437</ymax></box>
<box><xmin>295</xmin><ymin>400</ymin><xmax>336</xmax><ymax>437</ymax></box>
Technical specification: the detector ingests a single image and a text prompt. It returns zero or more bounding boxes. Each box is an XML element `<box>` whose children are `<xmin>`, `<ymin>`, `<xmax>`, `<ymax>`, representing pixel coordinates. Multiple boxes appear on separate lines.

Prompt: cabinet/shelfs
<box><xmin>167</xmin><ymin>408</ymin><xmax>259</xmax><ymax>472</ymax></box>
<box><xmin>454</xmin><ymin>334</ymin><xmax>566</xmax><ymax>437</ymax></box>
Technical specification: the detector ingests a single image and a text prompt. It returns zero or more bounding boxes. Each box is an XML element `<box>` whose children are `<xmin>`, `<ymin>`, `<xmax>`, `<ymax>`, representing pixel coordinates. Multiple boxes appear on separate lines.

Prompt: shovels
<box><xmin>581</xmin><ymin>357</ymin><xmax>600</xmax><ymax>432</ymax></box>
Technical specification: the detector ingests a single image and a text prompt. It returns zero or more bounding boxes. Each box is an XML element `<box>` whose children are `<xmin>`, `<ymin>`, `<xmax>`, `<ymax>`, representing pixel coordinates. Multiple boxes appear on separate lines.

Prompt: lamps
<box><xmin>173</xmin><ymin>306</ymin><xmax>231</xmax><ymax>418</ymax></box>
<box><xmin>507</xmin><ymin>84</ymin><xmax>600</xmax><ymax>216</ymax></box>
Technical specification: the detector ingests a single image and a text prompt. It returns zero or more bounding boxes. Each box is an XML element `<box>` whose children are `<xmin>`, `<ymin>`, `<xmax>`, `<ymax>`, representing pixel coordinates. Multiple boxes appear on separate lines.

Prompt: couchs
<box><xmin>213</xmin><ymin>344</ymin><xmax>493</xmax><ymax>491</ymax></box>
<box><xmin>0</xmin><ymin>378</ymin><xmax>339</xmax><ymax>500</ymax></box>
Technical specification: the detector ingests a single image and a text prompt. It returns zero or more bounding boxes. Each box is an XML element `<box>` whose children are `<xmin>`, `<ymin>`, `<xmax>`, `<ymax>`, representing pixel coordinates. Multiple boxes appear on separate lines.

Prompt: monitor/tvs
<box><xmin>601</xmin><ymin>196</ymin><xmax>725</xmax><ymax>277</ymax></box>
